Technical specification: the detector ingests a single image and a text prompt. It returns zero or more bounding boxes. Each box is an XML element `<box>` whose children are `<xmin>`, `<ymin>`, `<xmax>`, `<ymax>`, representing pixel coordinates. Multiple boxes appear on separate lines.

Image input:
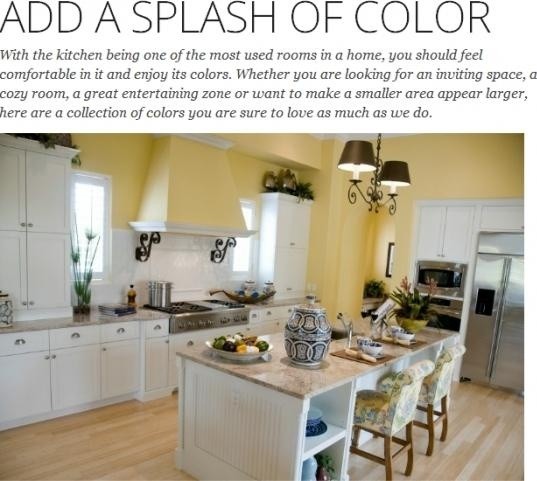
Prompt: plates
<box><xmin>205</xmin><ymin>339</ymin><xmax>273</xmax><ymax>361</ymax></box>
<box><xmin>305</xmin><ymin>421</ymin><xmax>327</xmax><ymax>437</ymax></box>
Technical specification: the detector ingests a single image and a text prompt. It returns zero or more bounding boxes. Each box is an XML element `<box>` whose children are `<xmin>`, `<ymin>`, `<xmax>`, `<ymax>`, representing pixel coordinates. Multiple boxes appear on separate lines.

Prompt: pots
<box><xmin>148</xmin><ymin>280</ymin><xmax>172</xmax><ymax>307</ymax></box>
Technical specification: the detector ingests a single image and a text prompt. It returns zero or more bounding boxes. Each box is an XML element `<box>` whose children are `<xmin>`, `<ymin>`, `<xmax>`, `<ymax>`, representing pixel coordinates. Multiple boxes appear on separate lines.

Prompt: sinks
<box><xmin>332</xmin><ymin>328</ymin><xmax>358</xmax><ymax>341</ymax></box>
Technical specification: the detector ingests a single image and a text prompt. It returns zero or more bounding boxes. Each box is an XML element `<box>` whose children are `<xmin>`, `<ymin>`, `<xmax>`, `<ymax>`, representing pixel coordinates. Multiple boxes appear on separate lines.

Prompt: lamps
<box><xmin>335</xmin><ymin>134</ymin><xmax>411</xmax><ymax>217</ymax></box>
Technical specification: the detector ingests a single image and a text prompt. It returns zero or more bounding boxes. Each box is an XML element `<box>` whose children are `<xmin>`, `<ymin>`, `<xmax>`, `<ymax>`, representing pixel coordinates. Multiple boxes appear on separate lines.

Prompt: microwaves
<box><xmin>416</xmin><ymin>260</ymin><xmax>468</xmax><ymax>298</ymax></box>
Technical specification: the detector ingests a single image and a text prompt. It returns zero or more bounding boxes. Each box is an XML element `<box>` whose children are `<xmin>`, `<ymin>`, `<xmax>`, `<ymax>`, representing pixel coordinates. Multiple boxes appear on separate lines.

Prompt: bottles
<box><xmin>244</xmin><ymin>280</ymin><xmax>255</xmax><ymax>296</ymax></box>
<box><xmin>284</xmin><ymin>303</ymin><xmax>332</xmax><ymax>366</ymax></box>
<box><xmin>263</xmin><ymin>282</ymin><xmax>274</xmax><ymax>304</ymax></box>
<box><xmin>0</xmin><ymin>292</ymin><xmax>13</xmax><ymax>327</ymax></box>
<box><xmin>127</xmin><ymin>284</ymin><xmax>137</xmax><ymax>307</ymax></box>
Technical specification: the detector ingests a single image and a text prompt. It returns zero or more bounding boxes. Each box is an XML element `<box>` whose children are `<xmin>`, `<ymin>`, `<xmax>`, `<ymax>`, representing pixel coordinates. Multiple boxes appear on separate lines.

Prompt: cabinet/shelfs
<box><xmin>295</xmin><ymin>381</ymin><xmax>350</xmax><ymax>481</ymax></box>
<box><xmin>413</xmin><ymin>197</ymin><xmax>524</xmax><ymax>263</ymax></box>
<box><xmin>0</xmin><ymin>133</ymin><xmax>312</xmax><ymax>433</ymax></box>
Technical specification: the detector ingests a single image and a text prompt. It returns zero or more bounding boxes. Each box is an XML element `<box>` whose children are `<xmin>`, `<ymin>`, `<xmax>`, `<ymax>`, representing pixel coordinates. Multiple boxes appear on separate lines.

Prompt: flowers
<box><xmin>384</xmin><ymin>277</ymin><xmax>441</xmax><ymax>325</ymax></box>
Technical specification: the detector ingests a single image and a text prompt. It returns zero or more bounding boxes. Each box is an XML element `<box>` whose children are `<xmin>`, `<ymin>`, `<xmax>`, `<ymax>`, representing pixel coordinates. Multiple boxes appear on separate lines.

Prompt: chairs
<box><xmin>350</xmin><ymin>360</ymin><xmax>435</xmax><ymax>481</ymax></box>
<box><xmin>376</xmin><ymin>342</ymin><xmax>466</xmax><ymax>455</ymax></box>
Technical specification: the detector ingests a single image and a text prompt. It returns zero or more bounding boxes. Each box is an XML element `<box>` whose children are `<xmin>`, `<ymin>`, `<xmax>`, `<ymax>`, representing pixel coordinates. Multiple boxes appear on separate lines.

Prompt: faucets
<box><xmin>336</xmin><ymin>312</ymin><xmax>353</xmax><ymax>350</ymax></box>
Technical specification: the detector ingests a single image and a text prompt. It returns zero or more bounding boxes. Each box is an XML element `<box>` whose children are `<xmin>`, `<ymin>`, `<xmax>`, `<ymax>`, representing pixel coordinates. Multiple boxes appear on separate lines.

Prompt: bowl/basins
<box><xmin>307</xmin><ymin>407</ymin><xmax>322</xmax><ymax>427</ymax></box>
<box><xmin>398</xmin><ymin>332</ymin><xmax>415</xmax><ymax>341</ymax></box>
<box><xmin>357</xmin><ymin>337</ymin><xmax>383</xmax><ymax>357</ymax></box>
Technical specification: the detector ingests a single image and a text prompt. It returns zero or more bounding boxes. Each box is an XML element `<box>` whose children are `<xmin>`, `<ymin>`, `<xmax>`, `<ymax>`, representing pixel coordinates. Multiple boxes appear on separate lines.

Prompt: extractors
<box><xmin>128</xmin><ymin>136</ymin><xmax>259</xmax><ymax>238</ymax></box>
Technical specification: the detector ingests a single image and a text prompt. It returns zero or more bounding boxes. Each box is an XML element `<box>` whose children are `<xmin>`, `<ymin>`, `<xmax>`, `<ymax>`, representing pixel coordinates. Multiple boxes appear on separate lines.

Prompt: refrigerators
<box><xmin>461</xmin><ymin>231</ymin><xmax>524</xmax><ymax>394</ymax></box>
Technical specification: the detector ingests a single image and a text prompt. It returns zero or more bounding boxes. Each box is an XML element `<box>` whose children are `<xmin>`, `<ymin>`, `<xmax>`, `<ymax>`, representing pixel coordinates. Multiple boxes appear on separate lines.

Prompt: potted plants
<box><xmin>365</xmin><ymin>280</ymin><xmax>385</xmax><ymax>298</ymax></box>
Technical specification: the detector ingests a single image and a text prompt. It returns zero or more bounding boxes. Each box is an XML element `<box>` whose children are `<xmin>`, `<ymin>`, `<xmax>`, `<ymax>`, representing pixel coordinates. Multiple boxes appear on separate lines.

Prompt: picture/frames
<box><xmin>386</xmin><ymin>242</ymin><xmax>395</xmax><ymax>277</ymax></box>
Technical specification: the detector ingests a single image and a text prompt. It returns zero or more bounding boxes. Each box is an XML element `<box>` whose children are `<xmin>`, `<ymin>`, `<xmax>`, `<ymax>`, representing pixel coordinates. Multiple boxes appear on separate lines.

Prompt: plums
<box><xmin>223</xmin><ymin>341</ymin><xmax>236</xmax><ymax>352</ymax></box>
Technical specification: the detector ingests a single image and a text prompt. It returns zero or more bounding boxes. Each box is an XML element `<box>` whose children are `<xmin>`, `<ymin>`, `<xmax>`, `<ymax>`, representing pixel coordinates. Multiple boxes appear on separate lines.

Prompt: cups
<box><xmin>391</xmin><ymin>325</ymin><xmax>404</xmax><ymax>338</ymax></box>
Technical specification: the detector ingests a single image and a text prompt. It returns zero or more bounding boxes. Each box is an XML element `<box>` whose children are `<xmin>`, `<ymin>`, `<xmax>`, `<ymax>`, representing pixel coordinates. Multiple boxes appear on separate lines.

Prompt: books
<box><xmin>99</xmin><ymin>304</ymin><xmax>136</xmax><ymax>317</ymax></box>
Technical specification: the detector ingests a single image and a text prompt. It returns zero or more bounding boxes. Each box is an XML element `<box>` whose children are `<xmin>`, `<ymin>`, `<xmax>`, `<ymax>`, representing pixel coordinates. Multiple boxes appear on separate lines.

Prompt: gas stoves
<box><xmin>143</xmin><ymin>299</ymin><xmax>250</xmax><ymax>334</ymax></box>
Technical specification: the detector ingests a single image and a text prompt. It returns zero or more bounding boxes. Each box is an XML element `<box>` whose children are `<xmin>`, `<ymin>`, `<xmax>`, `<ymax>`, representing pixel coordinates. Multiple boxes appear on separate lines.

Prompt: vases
<box><xmin>395</xmin><ymin>316</ymin><xmax>429</xmax><ymax>334</ymax></box>
